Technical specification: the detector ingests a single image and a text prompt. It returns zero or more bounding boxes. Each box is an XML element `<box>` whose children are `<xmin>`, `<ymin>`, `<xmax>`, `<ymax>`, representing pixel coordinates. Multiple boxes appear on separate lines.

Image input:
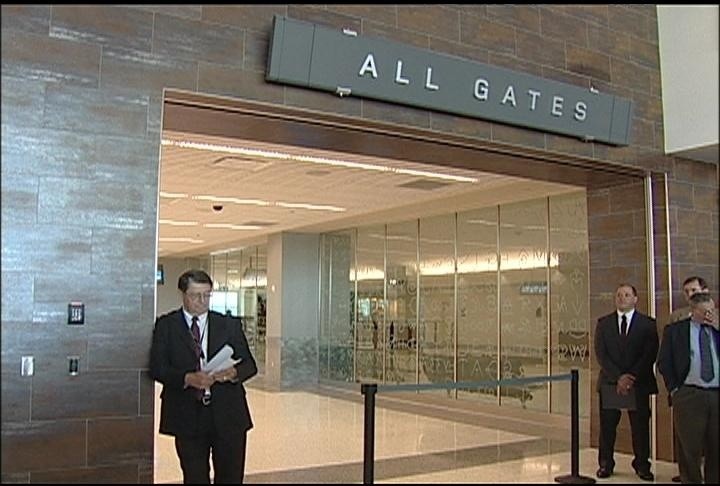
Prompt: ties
<box><xmin>698</xmin><ymin>324</ymin><xmax>714</xmax><ymax>383</ymax></box>
<box><xmin>190</xmin><ymin>317</ymin><xmax>206</xmax><ymax>402</ymax></box>
<box><xmin>619</xmin><ymin>315</ymin><xmax>627</xmax><ymax>350</ymax></box>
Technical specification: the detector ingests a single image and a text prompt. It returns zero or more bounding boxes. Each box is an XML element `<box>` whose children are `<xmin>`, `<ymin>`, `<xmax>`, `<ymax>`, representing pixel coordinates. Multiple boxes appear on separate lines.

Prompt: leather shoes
<box><xmin>672</xmin><ymin>477</ymin><xmax>681</xmax><ymax>482</ymax></box>
<box><xmin>597</xmin><ymin>464</ymin><xmax>615</xmax><ymax>478</ymax></box>
<box><xmin>632</xmin><ymin>466</ymin><xmax>654</xmax><ymax>481</ymax></box>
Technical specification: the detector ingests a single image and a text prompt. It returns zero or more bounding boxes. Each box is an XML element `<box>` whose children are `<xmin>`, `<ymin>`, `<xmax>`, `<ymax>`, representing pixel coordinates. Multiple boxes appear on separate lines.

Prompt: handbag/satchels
<box><xmin>600</xmin><ymin>383</ymin><xmax>639</xmax><ymax>411</ymax></box>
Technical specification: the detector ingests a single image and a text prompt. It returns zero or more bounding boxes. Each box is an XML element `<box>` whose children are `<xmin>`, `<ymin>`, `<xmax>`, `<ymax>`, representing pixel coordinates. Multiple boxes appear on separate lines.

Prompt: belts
<box><xmin>683</xmin><ymin>384</ymin><xmax>718</xmax><ymax>392</ymax></box>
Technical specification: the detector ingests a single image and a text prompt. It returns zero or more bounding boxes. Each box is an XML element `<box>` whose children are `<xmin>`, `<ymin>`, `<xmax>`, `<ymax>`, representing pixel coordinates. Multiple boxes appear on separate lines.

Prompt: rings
<box><xmin>627</xmin><ymin>384</ymin><xmax>630</xmax><ymax>389</ymax></box>
<box><xmin>224</xmin><ymin>375</ymin><xmax>227</xmax><ymax>381</ymax></box>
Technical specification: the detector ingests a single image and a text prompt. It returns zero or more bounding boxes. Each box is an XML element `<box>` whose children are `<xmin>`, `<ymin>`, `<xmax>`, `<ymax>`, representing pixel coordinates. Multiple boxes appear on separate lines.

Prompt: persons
<box><xmin>659</xmin><ymin>292</ymin><xmax>719</xmax><ymax>485</ymax></box>
<box><xmin>594</xmin><ymin>283</ymin><xmax>658</xmax><ymax>481</ymax></box>
<box><xmin>667</xmin><ymin>277</ymin><xmax>720</xmax><ymax>483</ymax></box>
<box><xmin>147</xmin><ymin>268</ymin><xmax>258</xmax><ymax>486</ymax></box>
